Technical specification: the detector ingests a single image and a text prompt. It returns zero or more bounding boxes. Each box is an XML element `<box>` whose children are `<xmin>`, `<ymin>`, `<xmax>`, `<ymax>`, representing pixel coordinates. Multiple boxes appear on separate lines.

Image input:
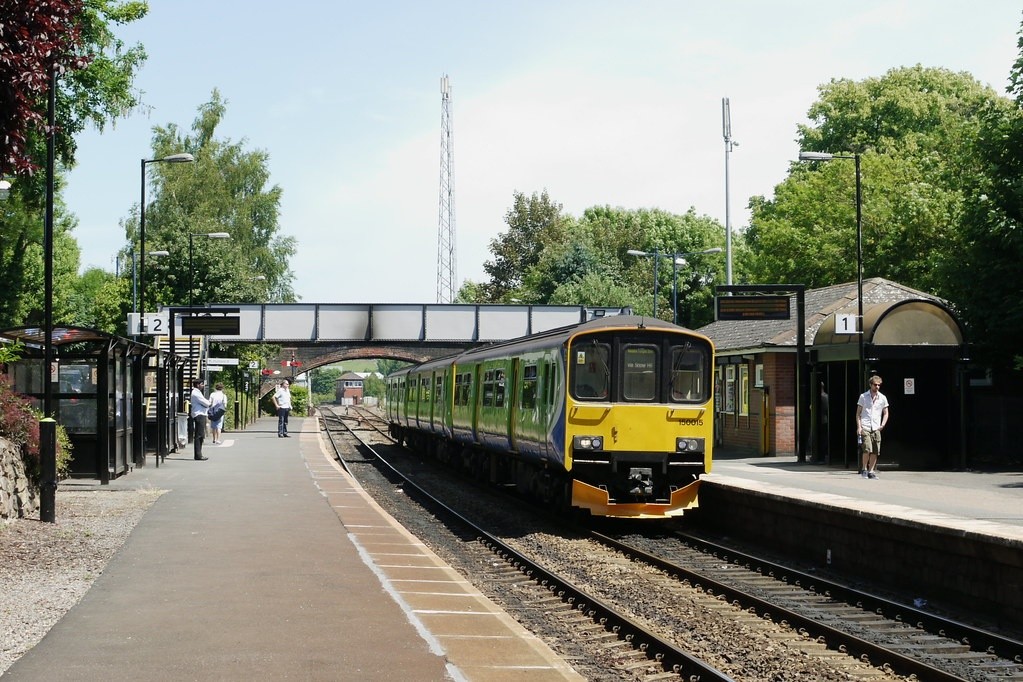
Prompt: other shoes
<box><xmin>194</xmin><ymin>456</ymin><xmax>208</xmax><ymax>460</ymax></box>
<box><xmin>861</xmin><ymin>469</ymin><xmax>869</xmax><ymax>479</ymax></box>
<box><xmin>215</xmin><ymin>441</ymin><xmax>222</xmax><ymax>444</ymax></box>
<box><xmin>868</xmin><ymin>471</ymin><xmax>879</xmax><ymax>480</ymax></box>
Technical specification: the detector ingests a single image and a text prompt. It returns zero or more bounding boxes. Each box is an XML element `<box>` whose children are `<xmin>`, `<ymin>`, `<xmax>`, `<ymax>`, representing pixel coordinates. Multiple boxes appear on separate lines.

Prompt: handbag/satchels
<box><xmin>207</xmin><ymin>399</ymin><xmax>225</xmax><ymax>422</ymax></box>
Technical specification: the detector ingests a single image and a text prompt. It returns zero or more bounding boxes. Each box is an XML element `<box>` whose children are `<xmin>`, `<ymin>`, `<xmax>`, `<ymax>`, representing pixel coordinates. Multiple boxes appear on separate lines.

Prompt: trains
<box><xmin>385</xmin><ymin>311</ymin><xmax>715</xmax><ymax>519</ymax></box>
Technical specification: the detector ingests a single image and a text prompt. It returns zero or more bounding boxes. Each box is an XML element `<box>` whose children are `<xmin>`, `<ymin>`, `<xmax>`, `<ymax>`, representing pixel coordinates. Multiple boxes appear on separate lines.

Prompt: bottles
<box><xmin>858</xmin><ymin>435</ymin><xmax>862</xmax><ymax>444</ymax></box>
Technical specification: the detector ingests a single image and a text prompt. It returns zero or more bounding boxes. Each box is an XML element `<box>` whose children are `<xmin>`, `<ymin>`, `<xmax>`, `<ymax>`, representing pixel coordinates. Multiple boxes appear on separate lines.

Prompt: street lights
<box><xmin>626</xmin><ymin>245</ymin><xmax>659</xmax><ymax>319</ymax></box>
<box><xmin>799</xmin><ymin>148</ymin><xmax>865</xmax><ymax>398</ymax></box>
<box><xmin>673</xmin><ymin>247</ymin><xmax>724</xmax><ymax>324</ymax></box>
<box><xmin>190</xmin><ymin>232</ymin><xmax>231</xmax><ymax>400</ymax></box>
<box><xmin>138</xmin><ymin>152</ymin><xmax>195</xmax><ymax>341</ymax></box>
<box><xmin>133</xmin><ymin>251</ymin><xmax>170</xmax><ymax>313</ymax></box>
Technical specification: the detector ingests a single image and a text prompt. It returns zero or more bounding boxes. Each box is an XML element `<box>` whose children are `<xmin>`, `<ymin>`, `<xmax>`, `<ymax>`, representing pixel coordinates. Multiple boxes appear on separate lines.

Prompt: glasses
<box><xmin>874</xmin><ymin>383</ymin><xmax>881</xmax><ymax>386</ymax></box>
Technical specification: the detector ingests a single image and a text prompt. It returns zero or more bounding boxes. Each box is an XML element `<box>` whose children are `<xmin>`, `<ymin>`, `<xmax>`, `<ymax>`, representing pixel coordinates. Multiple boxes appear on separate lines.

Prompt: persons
<box><xmin>272</xmin><ymin>380</ymin><xmax>293</xmax><ymax>438</ymax></box>
<box><xmin>191</xmin><ymin>379</ymin><xmax>211</xmax><ymax>460</ymax></box>
<box><xmin>209</xmin><ymin>383</ymin><xmax>227</xmax><ymax>444</ymax></box>
<box><xmin>856</xmin><ymin>376</ymin><xmax>889</xmax><ymax>479</ymax></box>
